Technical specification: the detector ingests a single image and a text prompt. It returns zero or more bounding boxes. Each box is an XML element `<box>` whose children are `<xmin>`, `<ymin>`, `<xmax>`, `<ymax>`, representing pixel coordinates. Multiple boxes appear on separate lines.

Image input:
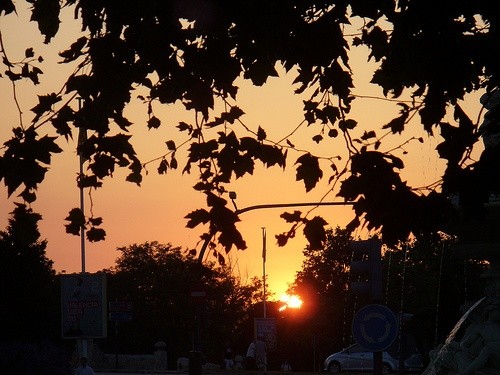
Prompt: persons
<box><xmin>225</xmin><ymin>336</ymin><xmax>268</xmax><ymax>375</ymax></box>
<box><xmin>281</xmin><ymin>361</ymin><xmax>292</xmax><ymax>375</ymax></box>
<box><xmin>62</xmin><ymin>278</ymin><xmax>83</xmax><ymax>336</ymax></box>
<box><xmin>75</xmin><ymin>356</ymin><xmax>96</xmax><ymax>375</ymax></box>
<box><xmin>431</xmin><ymin>288</ymin><xmax>500</xmax><ymax>375</ymax></box>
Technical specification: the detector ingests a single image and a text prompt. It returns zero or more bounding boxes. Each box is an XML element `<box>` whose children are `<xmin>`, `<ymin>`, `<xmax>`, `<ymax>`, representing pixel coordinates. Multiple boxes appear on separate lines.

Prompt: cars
<box><xmin>323</xmin><ymin>341</ymin><xmax>399</xmax><ymax>375</ymax></box>
<box><xmin>425</xmin><ymin>293</ymin><xmax>500</xmax><ymax>375</ymax></box>
